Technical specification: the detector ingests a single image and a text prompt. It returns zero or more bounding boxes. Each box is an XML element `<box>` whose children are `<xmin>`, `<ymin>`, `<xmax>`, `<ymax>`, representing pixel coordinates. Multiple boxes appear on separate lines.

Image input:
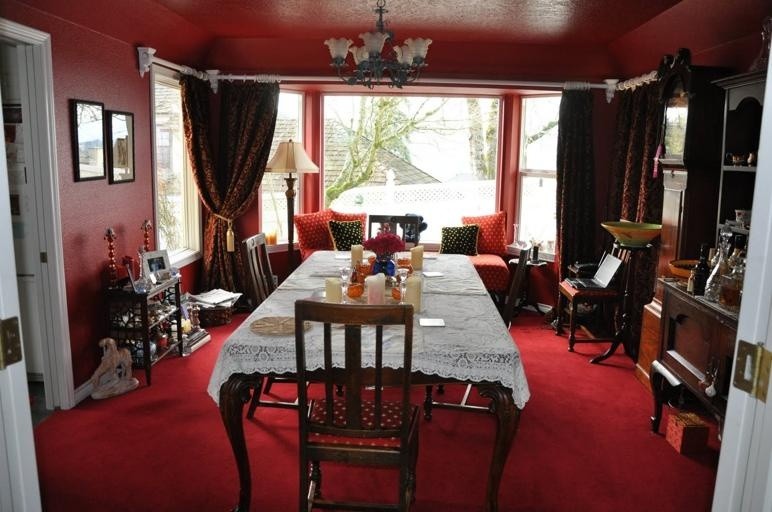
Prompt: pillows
<box><xmin>292</xmin><ymin>209</ymin><xmax>366</xmax><ymax>260</ymax></box>
<box><xmin>434</xmin><ymin>212</ymin><xmax>508</xmax><ymax>258</ymax></box>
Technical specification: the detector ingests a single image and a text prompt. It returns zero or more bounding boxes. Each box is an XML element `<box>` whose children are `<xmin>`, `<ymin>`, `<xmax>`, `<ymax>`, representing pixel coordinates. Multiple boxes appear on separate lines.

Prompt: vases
<box><xmin>368</xmin><ymin>254</ymin><xmax>395</xmax><ymax>276</ymax></box>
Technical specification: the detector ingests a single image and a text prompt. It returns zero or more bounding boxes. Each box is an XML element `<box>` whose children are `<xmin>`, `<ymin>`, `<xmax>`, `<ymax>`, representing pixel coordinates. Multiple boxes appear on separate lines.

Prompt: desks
<box><xmin>109</xmin><ymin>279</ymin><xmax>183</xmax><ymax>386</ymax></box>
<box><xmin>207</xmin><ymin>249</ymin><xmax>533</xmax><ymax>510</ymax></box>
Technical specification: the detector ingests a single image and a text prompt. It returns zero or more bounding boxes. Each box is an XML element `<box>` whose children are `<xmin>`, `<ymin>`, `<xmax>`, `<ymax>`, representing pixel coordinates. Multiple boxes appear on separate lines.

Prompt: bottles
<box><xmin>652</xmin><ymin>143</ymin><xmax>664</xmax><ymax>179</ymax></box>
<box><xmin>352</xmin><ymin>267</ymin><xmax>358</xmax><ymax>282</ymax></box>
<box><xmin>686</xmin><ymin>223</ymin><xmax>744</xmax><ymax>312</ymax></box>
<box><xmin>131</xmin><ymin>245</ymin><xmax>150</xmax><ymax>295</ymax></box>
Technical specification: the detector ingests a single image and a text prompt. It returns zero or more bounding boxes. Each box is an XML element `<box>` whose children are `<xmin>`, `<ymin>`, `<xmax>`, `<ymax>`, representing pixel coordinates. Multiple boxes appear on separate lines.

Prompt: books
<box><xmin>187</xmin><ymin>288</ymin><xmax>244</xmax><ymax>310</ymax></box>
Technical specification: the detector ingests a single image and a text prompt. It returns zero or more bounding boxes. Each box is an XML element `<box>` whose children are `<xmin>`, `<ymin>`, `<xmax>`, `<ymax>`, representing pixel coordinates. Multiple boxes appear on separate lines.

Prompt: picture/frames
<box><xmin>106</xmin><ymin>110</ymin><xmax>135</xmax><ymax>185</ymax></box>
<box><xmin>70</xmin><ymin>99</ymin><xmax>106</xmax><ymax>182</ymax></box>
<box><xmin>142</xmin><ymin>249</ymin><xmax>171</xmax><ymax>281</ymax></box>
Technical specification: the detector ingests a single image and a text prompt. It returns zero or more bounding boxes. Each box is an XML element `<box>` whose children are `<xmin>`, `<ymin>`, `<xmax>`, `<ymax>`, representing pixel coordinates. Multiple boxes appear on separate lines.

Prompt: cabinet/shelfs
<box><xmin>710</xmin><ymin>69</ymin><xmax>767</xmax><ymax>250</ymax></box>
<box><xmin>650</xmin><ymin>278</ymin><xmax>737</xmax><ymax>451</ymax></box>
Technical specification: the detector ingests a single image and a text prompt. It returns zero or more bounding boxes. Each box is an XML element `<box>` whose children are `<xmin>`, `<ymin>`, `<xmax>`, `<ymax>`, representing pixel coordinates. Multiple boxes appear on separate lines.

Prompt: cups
<box><xmin>735</xmin><ymin>209</ymin><xmax>751</xmax><ymax>225</ymax></box>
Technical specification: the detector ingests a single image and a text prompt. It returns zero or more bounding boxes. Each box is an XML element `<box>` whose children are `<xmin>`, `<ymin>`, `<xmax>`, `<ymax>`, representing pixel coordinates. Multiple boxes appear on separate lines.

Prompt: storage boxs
<box><xmin>665</xmin><ymin>415</ymin><xmax>710</xmax><ymax>455</ymax></box>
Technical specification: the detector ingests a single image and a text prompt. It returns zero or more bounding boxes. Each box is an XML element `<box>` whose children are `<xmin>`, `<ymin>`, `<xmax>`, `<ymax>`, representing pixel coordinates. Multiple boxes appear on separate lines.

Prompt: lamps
<box><xmin>324</xmin><ymin>2</ymin><xmax>432</xmax><ymax>90</ymax></box>
<box><xmin>261</xmin><ymin>138</ymin><xmax>320</xmax><ymax>269</ymax></box>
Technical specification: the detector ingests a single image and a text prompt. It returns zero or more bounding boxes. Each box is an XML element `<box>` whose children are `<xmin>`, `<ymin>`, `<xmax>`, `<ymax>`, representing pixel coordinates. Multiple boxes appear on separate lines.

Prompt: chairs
<box><xmin>553</xmin><ymin>245</ymin><xmax>636</xmax><ymax>352</ymax></box>
<box><xmin>294</xmin><ymin>300</ymin><xmax>420</xmax><ymax>512</ymax></box>
<box><xmin>242</xmin><ymin>232</ymin><xmax>308</xmax><ymax>426</ymax></box>
<box><xmin>368</xmin><ymin>216</ymin><xmax>420</xmax><ymax>252</ymax></box>
<box><xmin>424</xmin><ymin>243</ymin><xmax>534</xmax><ymax>419</ymax></box>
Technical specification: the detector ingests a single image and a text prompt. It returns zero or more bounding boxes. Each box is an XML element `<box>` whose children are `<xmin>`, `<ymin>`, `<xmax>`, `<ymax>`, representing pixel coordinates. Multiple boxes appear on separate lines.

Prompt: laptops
<box><xmin>565</xmin><ymin>253</ymin><xmax>624</xmax><ymax>290</ymax></box>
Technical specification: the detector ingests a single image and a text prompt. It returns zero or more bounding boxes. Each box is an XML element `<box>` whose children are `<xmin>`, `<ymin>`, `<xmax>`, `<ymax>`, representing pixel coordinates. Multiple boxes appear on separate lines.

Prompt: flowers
<box><xmin>364</xmin><ymin>234</ymin><xmax>403</xmax><ymax>252</ymax></box>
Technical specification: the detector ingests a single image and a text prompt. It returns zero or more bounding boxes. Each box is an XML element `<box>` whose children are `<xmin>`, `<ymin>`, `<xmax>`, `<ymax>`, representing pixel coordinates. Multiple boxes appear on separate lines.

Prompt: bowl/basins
<box><xmin>600</xmin><ymin>221</ymin><xmax>663</xmax><ymax>248</ymax></box>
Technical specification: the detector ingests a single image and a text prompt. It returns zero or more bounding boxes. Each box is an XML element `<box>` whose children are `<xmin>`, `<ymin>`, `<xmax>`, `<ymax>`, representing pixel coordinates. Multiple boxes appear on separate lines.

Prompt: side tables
<box><xmin>509</xmin><ymin>257</ymin><xmax>548</xmax><ymax>315</ymax></box>
